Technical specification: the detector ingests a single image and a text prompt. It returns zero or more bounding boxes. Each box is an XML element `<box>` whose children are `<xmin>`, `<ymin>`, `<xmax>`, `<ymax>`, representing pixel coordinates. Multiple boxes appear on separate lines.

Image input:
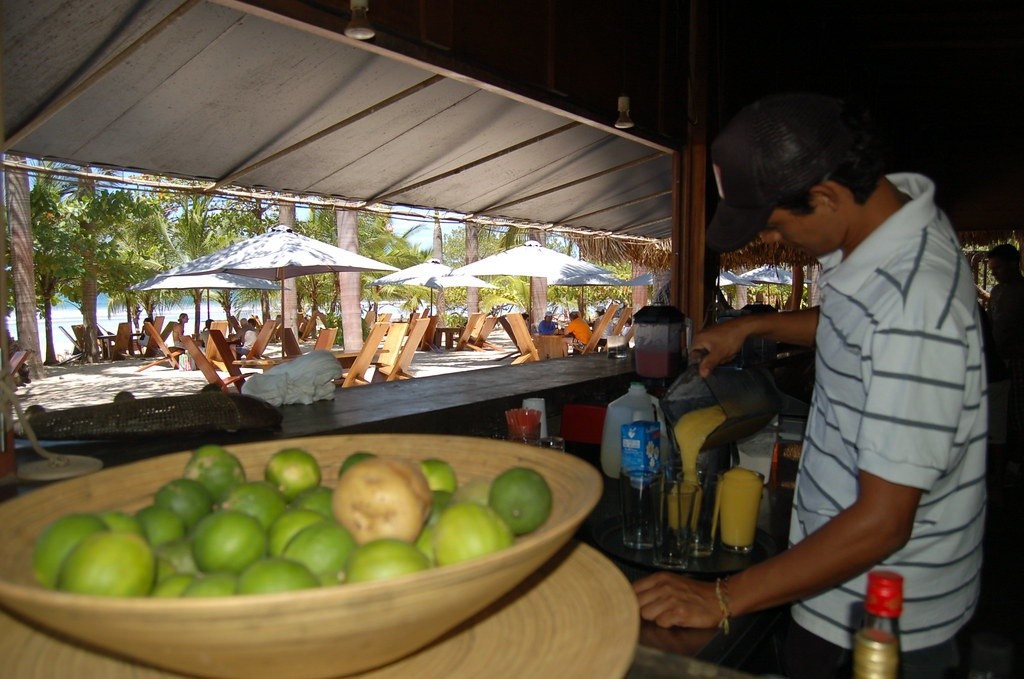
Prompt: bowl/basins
<box><xmin>0</xmin><ymin>435</ymin><xmax>604</xmax><ymax>679</ymax></box>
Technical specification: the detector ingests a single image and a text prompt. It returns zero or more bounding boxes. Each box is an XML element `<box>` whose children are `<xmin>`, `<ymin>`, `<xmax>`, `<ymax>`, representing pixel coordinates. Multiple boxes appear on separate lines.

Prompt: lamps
<box><xmin>343</xmin><ymin>0</ymin><xmax>376</xmax><ymax>42</ymax></box>
<box><xmin>612</xmin><ymin>88</ymin><xmax>637</xmax><ymax>130</ymax></box>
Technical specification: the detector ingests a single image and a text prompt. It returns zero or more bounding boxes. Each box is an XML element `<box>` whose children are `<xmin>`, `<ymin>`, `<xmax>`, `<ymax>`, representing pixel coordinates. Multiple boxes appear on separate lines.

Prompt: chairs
<box><xmin>10</xmin><ymin>350</ymin><xmax>29</xmax><ymax>376</ymax></box>
<box><xmin>59</xmin><ymin>303</ymin><xmax>634</xmax><ymax>394</ymax></box>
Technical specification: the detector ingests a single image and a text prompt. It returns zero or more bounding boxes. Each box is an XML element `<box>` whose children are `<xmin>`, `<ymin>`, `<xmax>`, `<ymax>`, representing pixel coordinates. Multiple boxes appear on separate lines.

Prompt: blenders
<box><xmin>633</xmin><ymin>303</ymin><xmax>777</xmax><ymax>421</ymax></box>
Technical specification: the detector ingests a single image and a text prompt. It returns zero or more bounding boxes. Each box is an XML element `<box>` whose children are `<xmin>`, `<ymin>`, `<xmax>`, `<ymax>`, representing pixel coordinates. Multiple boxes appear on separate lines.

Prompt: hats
<box><xmin>706</xmin><ymin>84</ymin><xmax>851</xmax><ymax>252</ymax></box>
<box><xmin>545</xmin><ymin>311</ymin><xmax>556</xmax><ymax>317</ymax></box>
<box><xmin>595</xmin><ymin>306</ymin><xmax>604</xmax><ymax>312</ymax></box>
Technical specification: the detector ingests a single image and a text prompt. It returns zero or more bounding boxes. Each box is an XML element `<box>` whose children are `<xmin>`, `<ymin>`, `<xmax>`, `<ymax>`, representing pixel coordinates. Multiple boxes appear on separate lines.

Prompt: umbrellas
<box><xmin>125</xmin><ymin>271</ymin><xmax>291</xmax><ymax>320</ymax></box>
<box><xmin>740</xmin><ymin>262</ymin><xmax>813</xmax><ymax>304</ymax></box>
<box><xmin>716</xmin><ymin>269</ymin><xmax>760</xmax><ymax>305</ymax></box>
<box><xmin>366</xmin><ymin>241</ymin><xmax>630</xmax><ymax>316</ymax></box>
<box><xmin>168</xmin><ymin>225</ymin><xmax>402</xmax><ymax>357</ymax></box>
<box><xmin>622</xmin><ymin>271</ymin><xmax>655</xmax><ymax>287</ymax></box>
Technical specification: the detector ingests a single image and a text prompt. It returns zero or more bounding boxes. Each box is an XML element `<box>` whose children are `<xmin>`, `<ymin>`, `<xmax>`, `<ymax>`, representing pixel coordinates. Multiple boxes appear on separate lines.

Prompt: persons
<box><xmin>521</xmin><ymin>303</ymin><xmax>633</xmax><ymax>355</ymax></box>
<box><xmin>747</xmin><ymin>290</ymin><xmax>764</xmax><ymax>305</ymax></box>
<box><xmin>978</xmin><ymin>244</ymin><xmax>1024</xmax><ymax>489</ymax></box>
<box><xmin>630</xmin><ymin>93</ymin><xmax>988</xmax><ymax>679</ymax></box>
<box><xmin>135</xmin><ymin>312</ymin><xmax>308</xmax><ymax>367</ymax></box>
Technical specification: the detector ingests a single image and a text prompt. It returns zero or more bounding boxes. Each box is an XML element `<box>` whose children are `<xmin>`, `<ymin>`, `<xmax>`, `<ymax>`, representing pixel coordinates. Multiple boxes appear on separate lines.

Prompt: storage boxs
<box><xmin>560</xmin><ymin>394</ymin><xmax>606</xmax><ymax>444</ymax></box>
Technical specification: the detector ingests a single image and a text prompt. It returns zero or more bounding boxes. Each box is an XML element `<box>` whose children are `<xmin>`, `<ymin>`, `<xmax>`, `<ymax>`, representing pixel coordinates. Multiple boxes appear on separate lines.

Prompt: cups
<box><xmin>523</xmin><ymin>398</ymin><xmax>547</xmax><ymax>439</ymax></box>
<box><xmin>503</xmin><ymin>423</ymin><xmax>541</xmax><ymax>448</ymax></box>
<box><xmin>607</xmin><ymin>335</ymin><xmax>628</xmax><ymax>359</ymax></box>
<box><xmin>677</xmin><ymin>471</ymin><xmax>724</xmax><ymax>557</ymax></box>
<box><xmin>718</xmin><ymin>469</ymin><xmax>765</xmax><ymax>554</ymax></box>
<box><xmin>619</xmin><ymin>464</ymin><xmax>664</xmax><ymax>551</ymax></box>
<box><xmin>650</xmin><ymin>480</ymin><xmax>703</xmax><ymax>569</ymax></box>
<box><xmin>540</xmin><ymin>437</ymin><xmax>564</xmax><ymax>450</ymax></box>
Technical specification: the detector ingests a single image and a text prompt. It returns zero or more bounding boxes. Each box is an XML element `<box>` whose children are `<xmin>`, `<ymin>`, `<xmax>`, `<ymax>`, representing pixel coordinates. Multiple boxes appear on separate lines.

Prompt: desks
<box><xmin>435</xmin><ymin>328</ymin><xmax>464</xmax><ymax>349</ymax></box>
<box><xmin>533</xmin><ymin>333</ymin><xmax>572</xmax><ymax>359</ymax></box>
<box><xmin>97</xmin><ymin>333</ymin><xmax>143</xmax><ymax>360</ymax></box>
<box><xmin>231</xmin><ymin>349</ymin><xmax>390</xmax><ymax>388</ymax></box>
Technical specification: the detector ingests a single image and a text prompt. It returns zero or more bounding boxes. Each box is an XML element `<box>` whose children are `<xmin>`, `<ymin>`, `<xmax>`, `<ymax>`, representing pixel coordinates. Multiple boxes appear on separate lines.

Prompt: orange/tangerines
<box><xmin>32</xmin><ymin>445</ymin><xmax>551</xmax><ymax>596</ymax></box>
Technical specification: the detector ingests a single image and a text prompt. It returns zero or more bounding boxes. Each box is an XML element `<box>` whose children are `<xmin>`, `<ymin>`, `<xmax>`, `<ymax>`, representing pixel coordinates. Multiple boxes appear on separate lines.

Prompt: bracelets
<box><xmin>714</xmin><ymin>575</ymin><xmax>731</xmax><ymax>636</ymax></box>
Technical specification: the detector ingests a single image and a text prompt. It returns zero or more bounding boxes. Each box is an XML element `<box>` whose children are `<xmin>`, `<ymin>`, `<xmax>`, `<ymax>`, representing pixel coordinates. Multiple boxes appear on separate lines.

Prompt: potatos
<box><xmin>331</xmin><ymin>455</ymin><xmax>433</xmax><ymax>543</ymax></box>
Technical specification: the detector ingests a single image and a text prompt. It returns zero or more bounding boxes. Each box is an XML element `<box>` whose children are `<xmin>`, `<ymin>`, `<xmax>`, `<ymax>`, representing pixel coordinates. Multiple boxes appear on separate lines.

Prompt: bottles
<box><xmin>601</xmin><ymin>382</ymin><xmax>654</xmax><ymax>478</ymax></box>
<box><xmin>620</xmin><ymin>410</ymin><xmax>661</xmax><ymax>479</ymax></box>
<box><xmin>861</xmin><ymin>571</ymin><xmax>905</xmax><ymax>639</ymax></box>
<box><xmin>849</xmin><ymin>629</ymin><xmax>900</xmax><ymax>679</ymax></box>
